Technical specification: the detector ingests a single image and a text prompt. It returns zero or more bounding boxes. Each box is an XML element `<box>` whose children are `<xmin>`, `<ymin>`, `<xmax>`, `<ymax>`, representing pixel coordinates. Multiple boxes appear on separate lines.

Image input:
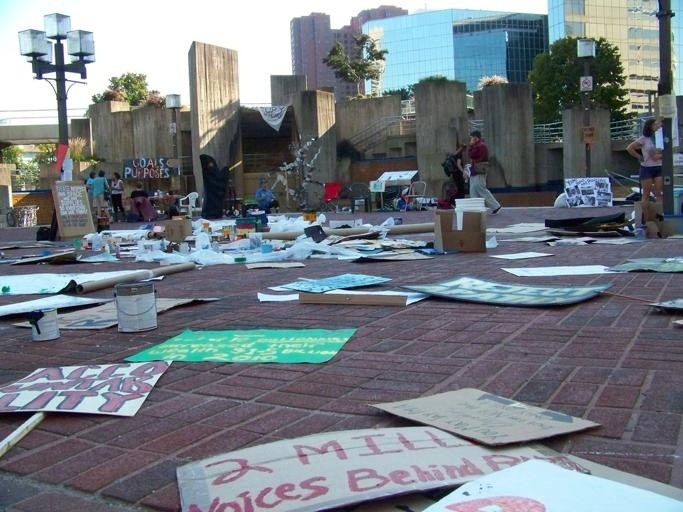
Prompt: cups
<box><xmin>142</xmin><ymin>241</ymin><xmax>190</xmax><ymax>254</ymax></box>
<box><xmin>4</xmin><ymin>203</ymin><xmax>40</xmax><ymax>227</ymax></box>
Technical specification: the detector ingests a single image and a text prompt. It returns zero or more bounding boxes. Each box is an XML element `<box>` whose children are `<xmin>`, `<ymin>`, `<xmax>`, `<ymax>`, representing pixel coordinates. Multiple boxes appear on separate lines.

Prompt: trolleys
<box><xmin>225</xmin><ymin>177</ymin><xmax>241</xmax><ymax>217</ymax></box>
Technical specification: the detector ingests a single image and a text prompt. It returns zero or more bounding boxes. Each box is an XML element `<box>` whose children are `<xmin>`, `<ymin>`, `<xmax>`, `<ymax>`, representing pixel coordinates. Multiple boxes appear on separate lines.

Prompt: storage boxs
<box><xmin>164</xmin><ymin>219</ymin><xmax>185</xmax><ymax>242</ymax></box>
<box><xmin>435</xmin><ymin>210</ymin><xmax>487</xmax><ymax>252</ymax></box>
<box><xmin>172</xmin><ymin>216</ymin><xmax>192</xmax><ymax>236</ymax></box>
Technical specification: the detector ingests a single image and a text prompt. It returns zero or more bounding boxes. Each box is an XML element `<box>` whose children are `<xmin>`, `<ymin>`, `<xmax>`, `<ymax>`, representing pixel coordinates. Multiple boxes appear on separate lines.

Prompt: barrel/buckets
<box><xmin>97</xmin><ymin>216</ymin><xmax>109</xmax><ymax>234</ymax></box>
<box><xmin>234</xmin><ymin>208</ymin><xmax>269</xmax><ymax>240</ymax></box>
<box><xmin>302</xmin><ymin>207</ymin><xmax>316</xmax><ymax>221</ymax></box>
<box><xmin>30</xmin><ymin>306</ymin><xmax>59</xmax><ymax>341</ymax></box>
<box><xmin>114</xmin><ymin>281</ymin><xmax>158</xmax><ymax>334</ymax></box>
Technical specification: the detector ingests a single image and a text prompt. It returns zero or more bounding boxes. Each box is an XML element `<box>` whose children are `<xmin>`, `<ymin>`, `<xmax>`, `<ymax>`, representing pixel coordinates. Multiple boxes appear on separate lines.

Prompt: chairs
<box><xmin>6</xmin><ymin>204</ymin><xmax>40</xmax><ymax>229</ymax></box>
<box><xmin>350</xmin><ymin>182</ymin><xmax>370</xmax><ymax>214</ymax></box>
<box><xmin>133</xmin><ymin>192</ymin><xmax>200</xmax><ymax>221</ymax></box>
<box><xmin>403</xmin><ymin>181</ymin><xmax>429</xmax><ymax>212</ymax></box>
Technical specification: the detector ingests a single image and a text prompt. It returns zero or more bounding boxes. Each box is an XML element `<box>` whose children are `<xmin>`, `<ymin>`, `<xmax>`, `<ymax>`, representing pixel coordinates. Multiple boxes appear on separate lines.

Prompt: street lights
<box><xmin>165</xmin><ymin>93</ymin><xmax>182</xmax><ymax>195</ymax></box>
<box><xmin>17</xmin><ymin>13</ymin><xmax>94</xmax><ymax>181</ymax></box>
<box><xmin>576</xmin><ymin>39</ymin><xmax>595</xmax><ymax>178</ymax></box>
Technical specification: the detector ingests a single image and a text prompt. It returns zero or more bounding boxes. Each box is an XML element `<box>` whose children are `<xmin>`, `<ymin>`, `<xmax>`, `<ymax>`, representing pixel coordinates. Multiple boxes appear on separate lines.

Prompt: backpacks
<box><xmin>441</xmin><ymin>151</ymin><xmax>460</xmax><ymax>179</ymax></box>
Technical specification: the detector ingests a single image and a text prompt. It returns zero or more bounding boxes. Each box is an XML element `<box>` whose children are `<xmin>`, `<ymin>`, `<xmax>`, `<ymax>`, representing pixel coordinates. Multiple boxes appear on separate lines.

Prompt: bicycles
<box><xmin>5</xmin><ymin>206</ymin><xmax>18</xmax><ymax>227</ymax></box>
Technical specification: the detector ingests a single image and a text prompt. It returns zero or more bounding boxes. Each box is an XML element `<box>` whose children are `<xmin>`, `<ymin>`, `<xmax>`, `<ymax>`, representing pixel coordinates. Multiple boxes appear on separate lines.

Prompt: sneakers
<box><xmin>489</xmin><ymin>206</ymin><xmax>503</xmax><ymax>216</ymax></box>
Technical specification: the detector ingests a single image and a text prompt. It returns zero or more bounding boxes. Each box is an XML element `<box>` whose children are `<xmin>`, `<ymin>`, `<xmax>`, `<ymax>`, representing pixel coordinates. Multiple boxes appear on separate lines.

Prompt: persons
<box><xmin>624</xmin><ymin>118</ymin><xmax>664</xmax><ymax>205</ymax></box>
<box><xmin>450</xmin><ymin>143</ymin><xmax>468</xmax><ymax>208</ymax></box>
<box><xmin>84</xmin><ymin>170</ymin><xmax>125</xmax><ymax>223</ymax></box>
<box><xmin>130</xmin><ymin>181</ymin><xmax>148</xmax><ymax>199</ymax></box>
<box><xmin>164</xmin><ymin>189</ymin><xmax>177</xmax><ymax>217</ymax></box>
<box><xmin>465</xmin><ymin>130</ymin><xmax>502</xmax><ymax>215</ymax></box>
<box><xmin>254</xmin><ymin>179</ymin><xmax>279</xmax><ymax>214</ymax></box>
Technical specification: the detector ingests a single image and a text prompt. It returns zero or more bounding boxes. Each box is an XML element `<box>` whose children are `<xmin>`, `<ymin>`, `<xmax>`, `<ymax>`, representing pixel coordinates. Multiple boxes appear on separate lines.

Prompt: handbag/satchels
<box><xmin>473</xmin><ymin>161</ymin><xmax>490</xmax><ymax>174</ymax></box>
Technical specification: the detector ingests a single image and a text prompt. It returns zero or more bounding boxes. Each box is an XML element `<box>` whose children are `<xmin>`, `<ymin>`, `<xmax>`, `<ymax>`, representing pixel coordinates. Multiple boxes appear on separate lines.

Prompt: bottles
<box><xmin>199</xmin><ymin>230</ymin><xmax>210</xmax><ymax>251</ymax></box>
<box><xmin>104</xmin><ymin>241</ymin><xmax>121</xmax><ymax>258</ymax></box>
